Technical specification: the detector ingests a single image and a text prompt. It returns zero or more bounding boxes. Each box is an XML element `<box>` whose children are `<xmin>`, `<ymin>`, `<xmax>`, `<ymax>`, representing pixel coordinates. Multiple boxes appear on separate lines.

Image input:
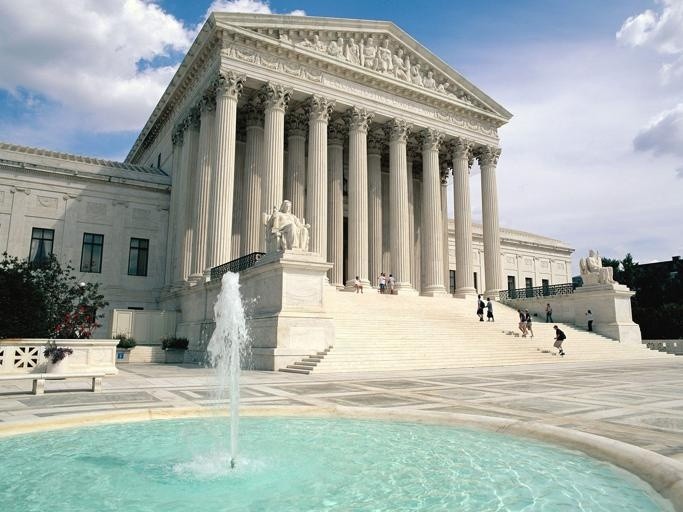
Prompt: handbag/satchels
<box><xmin>556</xmin><ymin>329</ymin><xmax>565</xmax><ymax>340</ymax></box>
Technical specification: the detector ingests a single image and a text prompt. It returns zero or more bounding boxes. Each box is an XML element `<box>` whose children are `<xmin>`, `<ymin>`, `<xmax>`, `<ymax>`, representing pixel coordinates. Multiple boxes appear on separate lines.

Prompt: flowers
<box><xmin>44</xmin><ymin>337</ymin><xmax>74</xmax><ymax>365</ymax></box>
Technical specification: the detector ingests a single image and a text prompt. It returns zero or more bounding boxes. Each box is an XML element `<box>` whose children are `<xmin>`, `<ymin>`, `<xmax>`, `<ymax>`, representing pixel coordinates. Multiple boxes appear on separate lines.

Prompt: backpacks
<box><xmin>480</xmin><ymin>301</ymin><xmax>484</xmax><ymax>308</ymax></box>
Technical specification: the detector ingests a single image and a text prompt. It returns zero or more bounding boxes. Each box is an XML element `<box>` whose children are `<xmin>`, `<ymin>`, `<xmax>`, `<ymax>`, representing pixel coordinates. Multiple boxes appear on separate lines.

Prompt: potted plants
<box><xmin>158</xmin><ymin>336</ymin><xmax>189</xmax><ymax>364</ymax></box>
<box><xmin>111</xmin><ymin>334</ymin><xmax>134</xmax><ymax>363</ymax></box>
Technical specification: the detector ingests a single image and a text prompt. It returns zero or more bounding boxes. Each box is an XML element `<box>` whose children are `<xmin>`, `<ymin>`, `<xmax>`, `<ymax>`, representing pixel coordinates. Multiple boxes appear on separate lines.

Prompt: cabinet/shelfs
<box><xmin>108</xmin><ymin>306</ymin><xmax>177</xmax><ymax>347</ymax></box>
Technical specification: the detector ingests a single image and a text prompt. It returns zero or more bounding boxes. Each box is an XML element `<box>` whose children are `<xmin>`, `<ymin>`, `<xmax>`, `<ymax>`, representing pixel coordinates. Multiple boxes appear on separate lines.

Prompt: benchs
<box><xmin>0</xmin><ymin>368</ymin><xmax>105</xmax><ymax>396</ymax></box>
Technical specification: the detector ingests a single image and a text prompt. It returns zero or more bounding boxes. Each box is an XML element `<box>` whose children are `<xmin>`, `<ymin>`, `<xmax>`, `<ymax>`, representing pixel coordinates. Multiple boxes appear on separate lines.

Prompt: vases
<box><xmin>46</xmin><ymin>357</ymin><xmax>65</xmax><ymax>374</ymax></box>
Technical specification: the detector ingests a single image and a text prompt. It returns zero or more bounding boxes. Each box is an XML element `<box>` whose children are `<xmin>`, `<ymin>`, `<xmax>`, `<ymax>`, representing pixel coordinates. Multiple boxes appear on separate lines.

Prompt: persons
<box><xmin>586</xmin><ymin>249</ymin><xmax>616</xmax><ymax>285</ymax></box>
<box><xmin>353</xmin><ymin>276</ymin><xmax>363</xmax><ymax>294</ymax></box>
<box><xmin>583</xmin><ymin>309</ymin><xmax>594</xmax><ymax>332</ymax></box>
<box><xmin>483</xmin><ymin>298</ymin><xmax>494</xmax><ymax>322</ymax></box>
<box><xmin>516</xmin><ymin>309</ymin><xmax>528</xmax><ymax>338</ymax></box>
<box><xmin>522</xmin><ymin>309</ymin><xmax>533</xmax><ymax>338</ymax></box>
<box><xmin>267</xmin><ymin>199</ymin><xmax>311</xmax><ymax>251</ymax></box>
<box><xmin>377</xmin><ymin>272</ymin><xmax>386</xmax><ymax>294</ymax></box>
<box><xmin>545</xmin><ymin>302</ymin><xmax>554</xmax><ymax>323</ymax></box>
<box><xmin>551</xmin><ymin>325</ymin><xmax>565</xmax><ymax>356</ymax></box>
<box><xmin>475</xmin><ymin>293</ymin><xmax>485</xmax><ymax>321</ymax></box>
<box><xmin>377</xmin><ymin>273</ymin><xmax>383</xmax><ymax>279</ymax></box>
<box><xmin>386</xmin><ymin>274</ymin><xmax>395</xmax><ymax>294</ymax></box>
